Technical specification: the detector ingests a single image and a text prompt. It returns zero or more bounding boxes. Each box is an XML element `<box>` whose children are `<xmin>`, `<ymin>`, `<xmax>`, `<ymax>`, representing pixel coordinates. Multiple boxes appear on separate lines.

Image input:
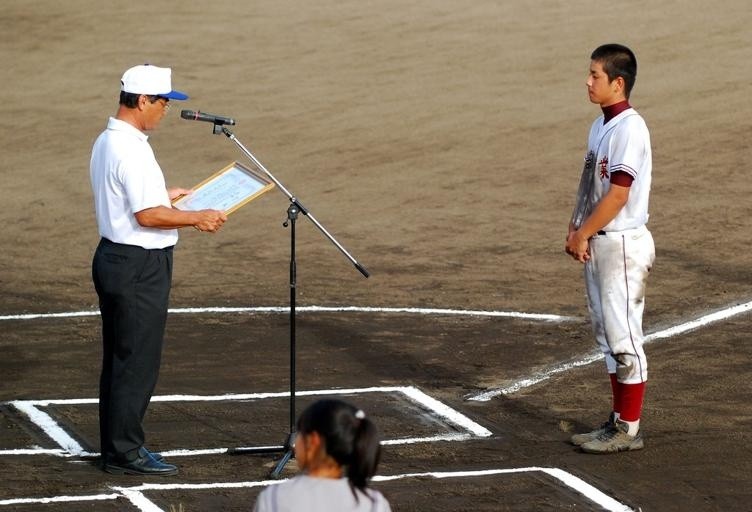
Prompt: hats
<box><xmin>121</xmin><ymin>65</ymin><xmax>188</xmax><ymax>101</ymax></box>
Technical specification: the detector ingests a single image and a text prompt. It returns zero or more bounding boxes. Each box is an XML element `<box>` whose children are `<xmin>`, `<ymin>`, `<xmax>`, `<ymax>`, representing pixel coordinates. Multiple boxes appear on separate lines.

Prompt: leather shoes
<box><xmin>104</xmin><ymin>446</ymin><xmax>179</xmax><ymax>477</ymax></box>
<box><xmin>145</xmin><ymin>447</ymin><xmax>168</xmax><ymax>464</ymax></box>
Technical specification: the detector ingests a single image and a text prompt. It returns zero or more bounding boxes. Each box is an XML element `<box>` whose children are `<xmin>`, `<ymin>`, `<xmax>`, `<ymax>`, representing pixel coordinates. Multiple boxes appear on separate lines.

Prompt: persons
<box><xmin>253</xmin><ymin>400</ymin><xmax>392</xmax><ymax>512</ymax></box>
<box><xmin>89</xmin><ymin>62</ymin><xmax>227</xmax><ymax>477</ymax></box>
<box><xmin>564</xmin><ymin>43</ymin><xmax>656</xmax><ymax>453</ymax></box>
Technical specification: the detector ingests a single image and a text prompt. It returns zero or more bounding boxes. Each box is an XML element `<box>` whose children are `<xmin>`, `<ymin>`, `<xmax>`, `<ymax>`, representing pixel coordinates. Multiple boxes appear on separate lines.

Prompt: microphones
<box><xmin>181</xmin><ymin>111</ymin><xmax>235</xmax><ymax>125</ymax></box>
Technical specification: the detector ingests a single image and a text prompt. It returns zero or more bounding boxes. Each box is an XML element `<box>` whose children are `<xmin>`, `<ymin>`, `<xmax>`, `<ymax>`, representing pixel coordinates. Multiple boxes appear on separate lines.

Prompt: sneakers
<box><xmin>580</xmin><ymin>417</ymin><xmax>643</xmax><ymax>455</ymax></box>
<box><xmin>570</xmin><ymin>410</ymin><xmax>615</xmax><ymax>446</ymax></box>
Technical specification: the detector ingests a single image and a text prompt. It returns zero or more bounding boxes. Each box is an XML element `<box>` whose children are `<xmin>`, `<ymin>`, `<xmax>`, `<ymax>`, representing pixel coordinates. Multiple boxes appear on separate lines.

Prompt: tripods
<box><xmin>213</xmin><ymin>117</ymin><xmax>370</xmax><ymax>479</ymax></box>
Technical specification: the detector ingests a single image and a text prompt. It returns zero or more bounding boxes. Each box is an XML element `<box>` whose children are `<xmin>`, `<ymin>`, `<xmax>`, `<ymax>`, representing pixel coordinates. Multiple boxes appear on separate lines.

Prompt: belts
<box><xmin>597</xmin><ymin>229</ymin><xmax>606</xmax><ymax>235</ymax></box>
